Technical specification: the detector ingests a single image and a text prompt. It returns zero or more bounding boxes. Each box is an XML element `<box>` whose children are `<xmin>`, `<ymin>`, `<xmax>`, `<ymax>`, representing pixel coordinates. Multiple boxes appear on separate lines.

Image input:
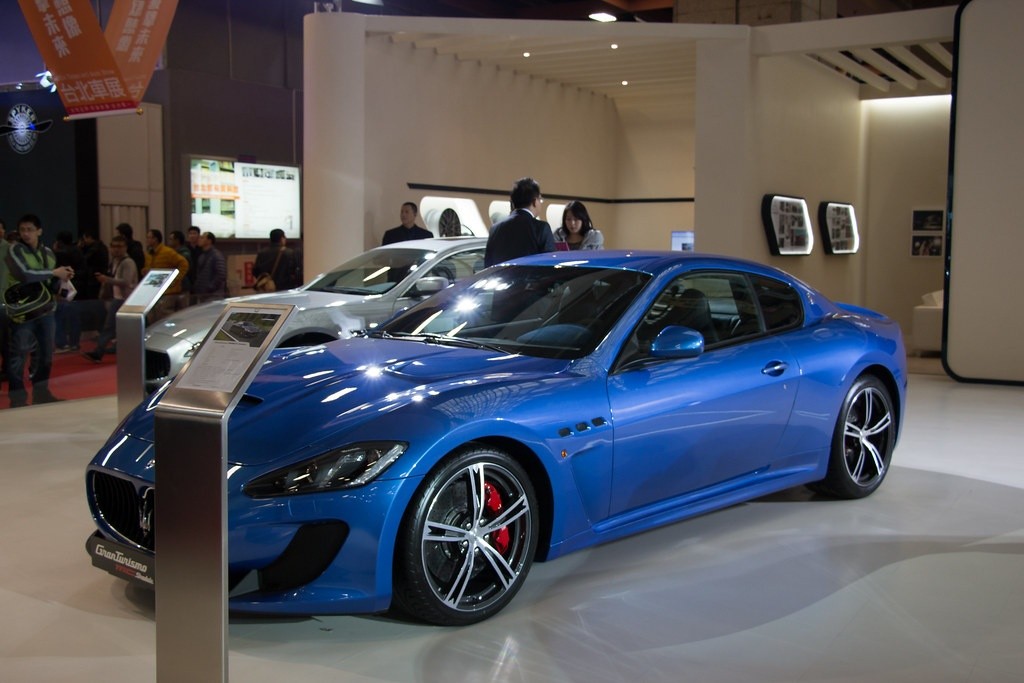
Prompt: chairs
<box><xmin>661</xmin><ymin>288</ymin><xmax>719</xmax><ymax>345</ymax></box>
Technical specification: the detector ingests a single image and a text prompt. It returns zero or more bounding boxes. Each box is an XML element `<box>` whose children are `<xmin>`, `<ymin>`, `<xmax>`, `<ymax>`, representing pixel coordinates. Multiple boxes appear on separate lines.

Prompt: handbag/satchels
<box><xmin>2</xmin><ymin>276</ymin><xmax>58</xmax><ymax>324</ymax></box>
<box><xmin>253</xmin><ymin>272</ymin><xmax>275</xmax><ymax>293</ymax></box>
<box><xmin>98</xmin><ymin>272</ymin><xmax>115</xmax><ymax>299</ymax></box>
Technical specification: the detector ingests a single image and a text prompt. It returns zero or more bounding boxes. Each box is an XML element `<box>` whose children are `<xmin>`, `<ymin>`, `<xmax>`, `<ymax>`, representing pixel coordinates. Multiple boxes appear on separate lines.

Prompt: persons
<box><xmin>831</xmin><ymin>207</ymin><xmax>851</xmax><ymax>251</ymax></box>
<box><xmin>780</xmin><ymin>203</ymin><xmax>807</xmax><ymax>247</ymax></box>
<box><xmin>188</xmin><ymin>227</ymin><xmax>202</xmax><ymax>306</ymax></box>
<box><xmin>197</xmin><ymin>232</ymin><xmax>226</xmax><ymax>302</ymax></box>
<box><xmin>381</xmin><ymin>202</ymin><xmax>434</xmax><ymax>282</ymax></box>
<box><xmin>141</xmin><ymin>229</ymin><xmax>189</xmax><ymax>325</ymax></box>
<box><xmin>0</xmin><ymin>215</ymin><xmax>75</xmax><ymax>408</ymax></box>
<box><xmin>167</xmin><ymin>231</ymin><xmax>191</xmax><ymax>311</ymax></box>
<box><xmin>82</xmin><ymin>236</ymin><xmax>137</xmax><ymax>362</ymax></box>
<box><xmin>920</xmin><ymin>240</ymin><xmax>929</xmax><ymax>255</ymax></box>
<box><xmin>554</xmin><ymin>201</ymin><xmax>604</xmax><ymax>322</ymax></box>
<box><xmin>251</xmin><ymin>229</ymin><xmax>299</xmax><ymax>291</ymax></box>
<box><xmin>52</xmin><ymin>223</ymin><xmax>145</xmax><ymax>354</ymax></box>
<box><xmin>485</xmin><ymin>178</ymin><xmax>556</xmax><ymax>323</ymax></box>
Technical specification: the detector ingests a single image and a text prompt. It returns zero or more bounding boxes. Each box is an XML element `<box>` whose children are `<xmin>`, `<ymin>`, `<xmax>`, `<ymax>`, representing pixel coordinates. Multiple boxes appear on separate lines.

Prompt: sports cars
<box><xmin>85</xmin><ymin>236</ymin><xmax>907</xmax><ymax>627</ymax></box>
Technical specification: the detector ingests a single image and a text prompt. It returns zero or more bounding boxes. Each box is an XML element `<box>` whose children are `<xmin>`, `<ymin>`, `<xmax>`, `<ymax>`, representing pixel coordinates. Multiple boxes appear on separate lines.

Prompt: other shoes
<box><xmin>32</xmin><ymin>390</ymin><xmax>65</xmax><ymax>404</ymax></box>
<box><xmin>82</xmin><ymin>351</ymin><xmax>101</xmax><ymax>364</ymax></box>
<box><xmin>10</xmin><ymin>399</ymin><xmax>29</xmax><ymax>407</ymax></box>
<box><xmin>71</xmin><ymin>344</ymin><xmax>80</xmax><ymax>352</ymax></box>
<box><xmin>90</xmin><ymin>335</ymin><xmax>97</xmax><ymax>339</ymax></box>
<box><xmin>55</xmin><ymin>344</ymin><xmax>70</xmax><ymax>353</ymax></box>
<box><xmin>105</xmin><ymin>347</ymin><xmax>116</xmax><ymax>352</ymax></box>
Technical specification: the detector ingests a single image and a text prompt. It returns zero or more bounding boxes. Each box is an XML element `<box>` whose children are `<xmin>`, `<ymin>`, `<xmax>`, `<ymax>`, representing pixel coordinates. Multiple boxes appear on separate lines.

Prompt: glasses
<box><xmin>111</xmin><ymin>245</ymin><xmax>126</xmax><ymax>249</ymax></box>
<box><xmin>537</xmin><ymin>197</ymin><xmax>544</xmax><ymax>204</ymax></box>
